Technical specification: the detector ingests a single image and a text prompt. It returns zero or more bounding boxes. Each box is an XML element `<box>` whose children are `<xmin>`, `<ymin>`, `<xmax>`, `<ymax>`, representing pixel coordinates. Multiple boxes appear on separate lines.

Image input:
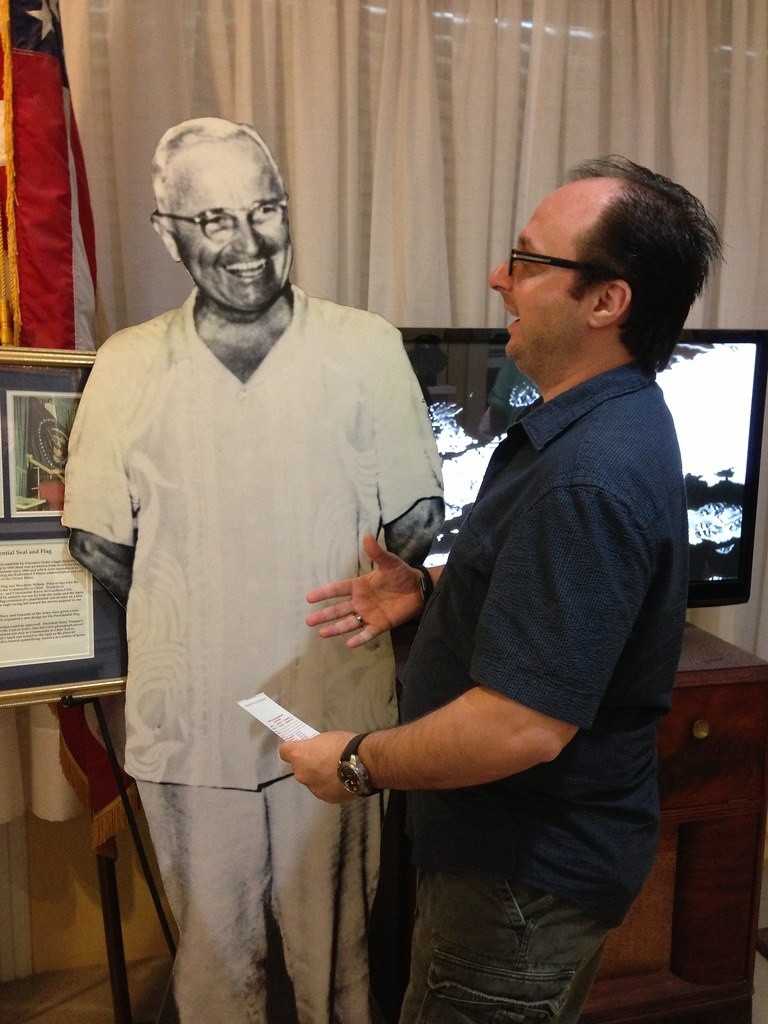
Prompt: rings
<box><xmin>352</xmin><ymin>612</ymin><xmax>364</xmax><ymax>625</ymax></box>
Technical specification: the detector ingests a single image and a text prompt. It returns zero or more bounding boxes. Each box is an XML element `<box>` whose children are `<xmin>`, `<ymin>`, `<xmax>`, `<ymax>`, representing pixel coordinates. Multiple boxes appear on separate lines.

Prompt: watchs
<box><xmin>337</xmin><ymin>732</ymin><xmax>385</xmax><ymax>797</ymax></box>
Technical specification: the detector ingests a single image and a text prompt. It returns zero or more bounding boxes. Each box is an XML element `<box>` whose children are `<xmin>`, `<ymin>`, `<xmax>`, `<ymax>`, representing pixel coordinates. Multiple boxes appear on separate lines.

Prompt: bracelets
<box><xmin>417</xmin><ymin>565</ymin><xmax>434</xmax><ymax>607</ymax></box>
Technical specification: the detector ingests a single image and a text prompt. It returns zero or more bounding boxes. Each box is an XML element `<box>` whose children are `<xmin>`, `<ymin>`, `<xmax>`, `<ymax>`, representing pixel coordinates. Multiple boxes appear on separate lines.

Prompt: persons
<box><xmin>61</xmin><ymin>118</ymin><xmax>446</xmax><ymax>1024</ymax></box>
<box><xmin>278</xmin><ymin>156</ymin><xmax>726</xmax><ymax>1024</ymax></box>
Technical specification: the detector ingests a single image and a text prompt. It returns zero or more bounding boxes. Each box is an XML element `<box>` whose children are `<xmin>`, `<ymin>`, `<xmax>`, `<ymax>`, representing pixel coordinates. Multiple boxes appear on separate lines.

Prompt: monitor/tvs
<box><xmin>397</xmin><ymin>328</ymin><xmax>768</xmax><ymax>609</ymax></box>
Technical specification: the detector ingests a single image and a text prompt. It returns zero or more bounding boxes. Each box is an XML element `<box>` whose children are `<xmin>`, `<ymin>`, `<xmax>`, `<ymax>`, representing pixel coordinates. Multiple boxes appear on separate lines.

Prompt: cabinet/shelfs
<box><xmin>579</xmin><ymin>624</ymin><xmax>767</xmax><ymax>1024</ymax></box>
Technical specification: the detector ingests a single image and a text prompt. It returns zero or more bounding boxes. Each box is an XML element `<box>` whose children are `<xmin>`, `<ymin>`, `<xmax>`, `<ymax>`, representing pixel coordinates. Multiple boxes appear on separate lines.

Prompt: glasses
<box><xmin>152</xmin><ymin>191</ymin><xmax>291</xmax><ymax>244</ymax></box>
<box><xmin>506</xmin><ymin>245</ymin><xmax>624</xmax><ymax>283</ymax></box>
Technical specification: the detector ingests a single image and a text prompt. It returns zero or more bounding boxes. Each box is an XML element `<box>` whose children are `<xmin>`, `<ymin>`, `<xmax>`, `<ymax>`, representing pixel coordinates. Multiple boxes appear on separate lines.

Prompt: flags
<box><xmin>0</xmin><ymin>0</ymin><xmax>141</xmax><ymax>863</ymax></box>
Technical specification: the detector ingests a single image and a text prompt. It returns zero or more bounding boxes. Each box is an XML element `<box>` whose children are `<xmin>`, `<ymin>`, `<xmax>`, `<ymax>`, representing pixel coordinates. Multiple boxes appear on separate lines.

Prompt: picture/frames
<box><xmin>0</xmin><ymin>346</ymin><xmax>129</xmax><ymax>706</ymax></box>
